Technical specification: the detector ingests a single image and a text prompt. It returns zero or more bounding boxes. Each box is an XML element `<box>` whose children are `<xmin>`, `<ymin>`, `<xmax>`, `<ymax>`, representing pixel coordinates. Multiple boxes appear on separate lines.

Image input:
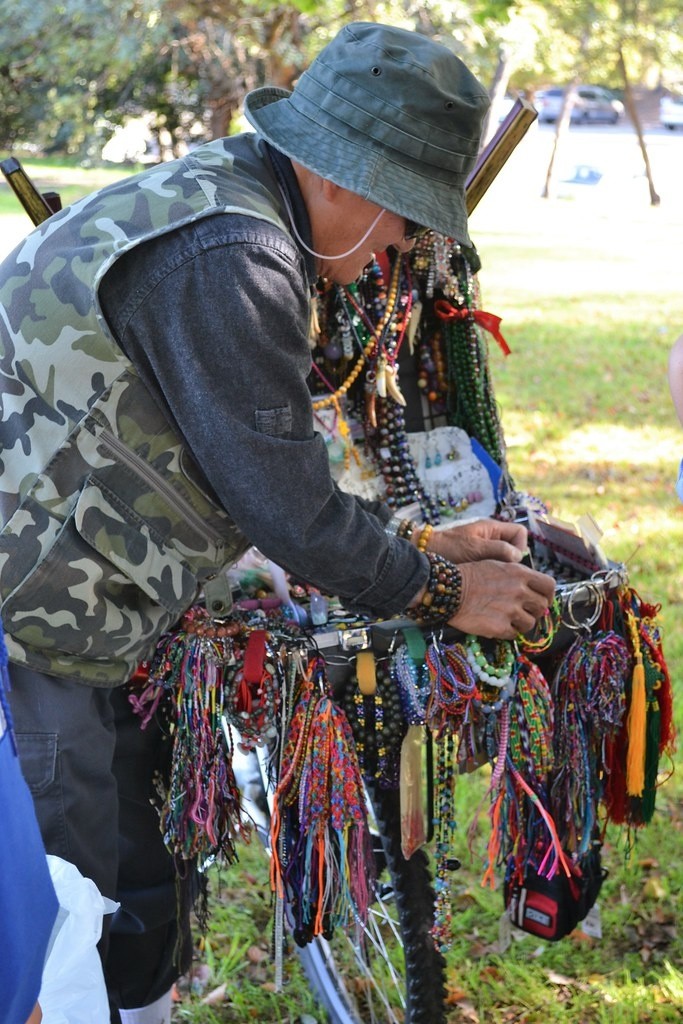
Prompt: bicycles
<box><xmin>170</xmin><ymin>573</ymin><xmax>461</xmax><ymax>1024</ymax></box>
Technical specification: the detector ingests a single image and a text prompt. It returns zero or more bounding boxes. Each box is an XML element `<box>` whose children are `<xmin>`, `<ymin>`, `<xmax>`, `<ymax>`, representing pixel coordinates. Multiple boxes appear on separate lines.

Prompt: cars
<box><xmin>659</xmin><ymin>90</ymin><xmax>683</xmax><ymax>129</ymax></box>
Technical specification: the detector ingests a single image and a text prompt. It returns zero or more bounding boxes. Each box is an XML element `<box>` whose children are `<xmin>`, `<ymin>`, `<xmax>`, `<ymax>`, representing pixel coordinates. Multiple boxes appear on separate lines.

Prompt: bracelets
<box><xmin>129</xmin><ymin>584</ymin><xmax>672</xmax><ymax>953</ymax></box>
<box><xmin>397</xmin><ymin>518</ymin><xmax>432</xmax><ymax>553</ymax></box>
<box><xmin>403</xmin><ymin>551</ymin><xmax>462</xmax><ymax>627</ymax></box>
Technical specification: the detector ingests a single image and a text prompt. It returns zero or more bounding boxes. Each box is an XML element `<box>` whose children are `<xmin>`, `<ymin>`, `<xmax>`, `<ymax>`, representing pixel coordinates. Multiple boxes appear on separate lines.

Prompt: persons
<box><xmin>668</xmin><ymin>333</ymin><xmax>683</xmax><ymax>505</ymax></box>
<box><xmin>0</xmin><ymin>22</ymin><xmax>557</xmax><ymax>1024</ymax></box>
<box><xmin>0</xmin><ymin>636</ymin><xmax>62</xmax><ymax>1024</ymax></box>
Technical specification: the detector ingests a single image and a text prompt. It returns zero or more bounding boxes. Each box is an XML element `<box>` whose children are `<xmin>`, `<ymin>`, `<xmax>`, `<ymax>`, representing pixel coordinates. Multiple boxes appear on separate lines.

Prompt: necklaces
<box><xmin>312</xmin><ymin>228</ymin><xmax>522</xmax><ymax>527</ymax></box>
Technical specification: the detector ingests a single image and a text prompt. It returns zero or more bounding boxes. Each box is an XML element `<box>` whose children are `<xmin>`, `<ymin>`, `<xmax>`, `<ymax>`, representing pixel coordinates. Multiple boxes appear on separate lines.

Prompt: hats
<box><xmin>242</xmin><ymin>21</ymin><xmax>491</xmax><ymax>249</ymax></box>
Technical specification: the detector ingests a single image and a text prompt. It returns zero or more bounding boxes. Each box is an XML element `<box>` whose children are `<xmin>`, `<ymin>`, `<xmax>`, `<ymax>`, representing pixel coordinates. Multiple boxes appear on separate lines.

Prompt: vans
<box><xmin>535</xmin><ymin>82</ymin><xmax>619</xmax><ymax>124</ymax></box>
